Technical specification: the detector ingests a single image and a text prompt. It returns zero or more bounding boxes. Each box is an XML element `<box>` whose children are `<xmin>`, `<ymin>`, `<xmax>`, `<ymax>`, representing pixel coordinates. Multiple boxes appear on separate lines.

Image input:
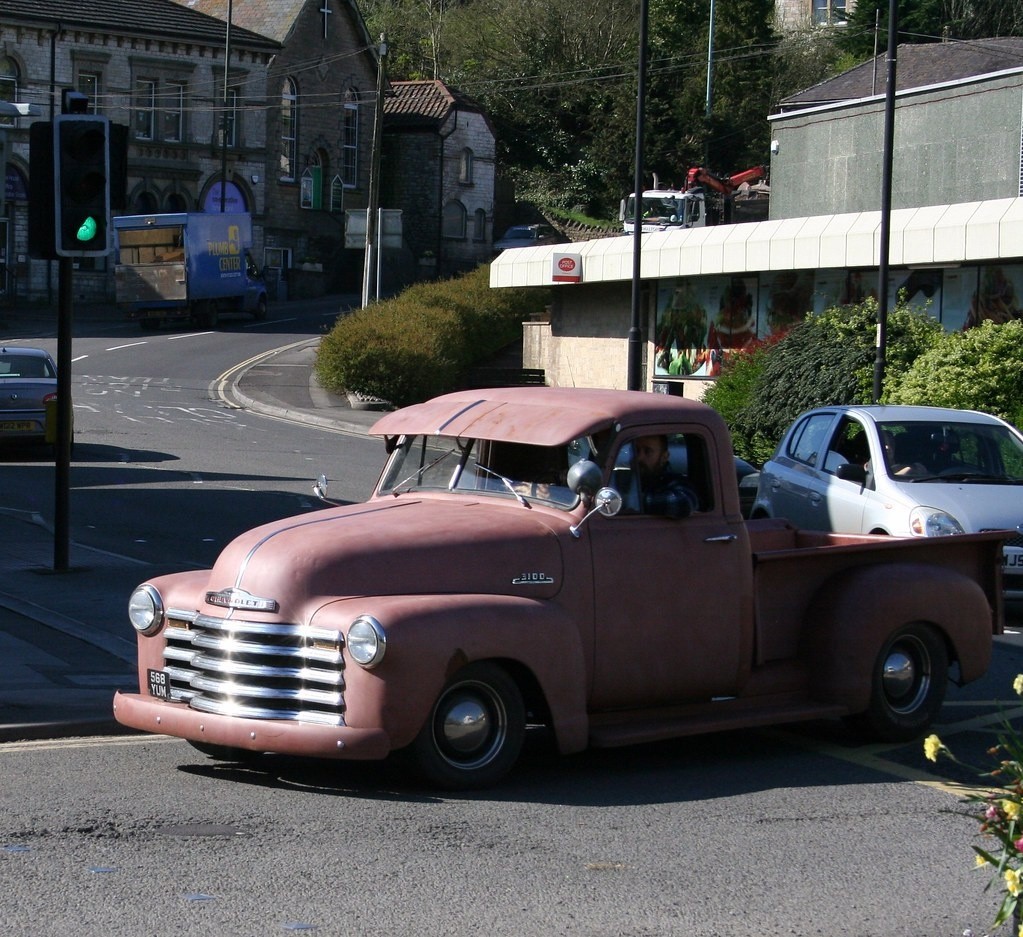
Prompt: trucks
<box><xmin>113</xmin><ymin>215</ymin><xmax>271</xmax><ymax>320</ymax></box>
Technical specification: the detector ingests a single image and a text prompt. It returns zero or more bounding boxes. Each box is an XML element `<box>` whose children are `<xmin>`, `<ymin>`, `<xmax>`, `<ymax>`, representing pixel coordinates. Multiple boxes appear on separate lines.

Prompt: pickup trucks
<box><xmin>113</xmin><ymin>387</ymin><xmax>1016</xmax><ymax>788</ymax></box>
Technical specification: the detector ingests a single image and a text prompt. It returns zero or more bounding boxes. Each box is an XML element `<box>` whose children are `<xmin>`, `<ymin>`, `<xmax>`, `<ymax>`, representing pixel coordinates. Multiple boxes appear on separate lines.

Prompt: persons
<box><xmin>536</xmin><ymin>434</ymin><xmax>699</xmax><ymax>515</ymax></box>
<box><xmin>864</xmin><ymin>430</ymin><xmax>921</xmax><ymax>474</ymax></box>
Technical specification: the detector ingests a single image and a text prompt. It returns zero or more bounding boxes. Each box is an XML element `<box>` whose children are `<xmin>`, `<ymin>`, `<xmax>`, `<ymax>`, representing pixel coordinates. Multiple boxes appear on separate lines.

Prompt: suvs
<box><xmin>750</xmin><ymin>405</ymin><xmax>1023</xmax><ymax>599</ymax></box>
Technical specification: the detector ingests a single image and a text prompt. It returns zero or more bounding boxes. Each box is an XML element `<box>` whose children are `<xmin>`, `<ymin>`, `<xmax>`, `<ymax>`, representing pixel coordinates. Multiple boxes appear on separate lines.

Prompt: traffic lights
<box><xmin>52</xmin><ymin>116</ymin><xmax>111</xmax><ymax>256</ymax></box>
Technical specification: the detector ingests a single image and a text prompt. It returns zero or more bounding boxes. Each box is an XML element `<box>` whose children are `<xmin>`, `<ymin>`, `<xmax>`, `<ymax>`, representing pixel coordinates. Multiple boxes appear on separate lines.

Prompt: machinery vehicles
<box><xmin>618</xmin><ymin>164</ymin><xmax>770</xmax><ymax>235</ymax></box>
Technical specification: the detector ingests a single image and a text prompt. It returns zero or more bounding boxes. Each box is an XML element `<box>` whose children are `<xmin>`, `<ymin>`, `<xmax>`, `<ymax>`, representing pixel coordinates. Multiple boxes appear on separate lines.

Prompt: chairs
<box><xmin>895</xmin><ymin>433</ymin><xmax>925</xmax><ymax>465</ymax></box>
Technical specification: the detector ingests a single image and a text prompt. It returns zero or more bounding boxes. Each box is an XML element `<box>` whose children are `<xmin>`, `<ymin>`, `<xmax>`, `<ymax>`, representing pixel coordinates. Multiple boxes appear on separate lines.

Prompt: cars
<box><xmin>493</xmin><ymin>223</ymin><xmax>558</xmax><ymax>250</ymax></box>
<box><xmin>0</xmin><ymin>346</ymin><xmax>74</xmax><ymax>453</ymax></box>
<box><xmin>562</xmin><ymin>436</ymin><xmax>760</xmax><ymax>520</ymax></box>
